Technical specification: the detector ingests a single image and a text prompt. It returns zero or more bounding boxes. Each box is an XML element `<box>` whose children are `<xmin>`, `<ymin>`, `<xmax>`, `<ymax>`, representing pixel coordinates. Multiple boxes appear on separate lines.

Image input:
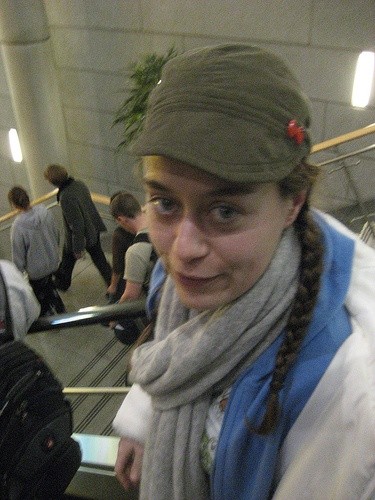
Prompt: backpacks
<box><xmin>0</xmin><ymin>274</ymin><xmax>83</xmax><ymax>500</ymax></box>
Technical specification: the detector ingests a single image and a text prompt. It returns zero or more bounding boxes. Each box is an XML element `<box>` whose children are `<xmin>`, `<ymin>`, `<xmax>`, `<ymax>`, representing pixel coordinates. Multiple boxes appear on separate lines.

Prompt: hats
<box><xmin>130</xmin><ymin>43</ymin><xmax>313</xmax><ymax>182</ymax></box>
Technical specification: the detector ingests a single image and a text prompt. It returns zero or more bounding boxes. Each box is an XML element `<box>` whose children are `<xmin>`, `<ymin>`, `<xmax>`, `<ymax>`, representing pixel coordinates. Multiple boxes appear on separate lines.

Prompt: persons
<box><xmin>105</xmin><ymin>191</ymin><xmax>162</xmax><ymax>335</ymax></box>
<box><xmin>7</xmin><ymin>187</ymin><xmax>67</xmax><ymax>314</ymax></box>
<box><xmin>111</xmin><ymin>42</ymin><xmax>375</xmax><ymax>500</ymax></box>
<box><xmin>0</xmin><ymin>261</ymin><xmax>40</xmax><ymax>347</ymax></box>
<box><xmin>42</xmin><ymin>164</ymin><xmax>112</xmax><ymax>293</ymax></box>
